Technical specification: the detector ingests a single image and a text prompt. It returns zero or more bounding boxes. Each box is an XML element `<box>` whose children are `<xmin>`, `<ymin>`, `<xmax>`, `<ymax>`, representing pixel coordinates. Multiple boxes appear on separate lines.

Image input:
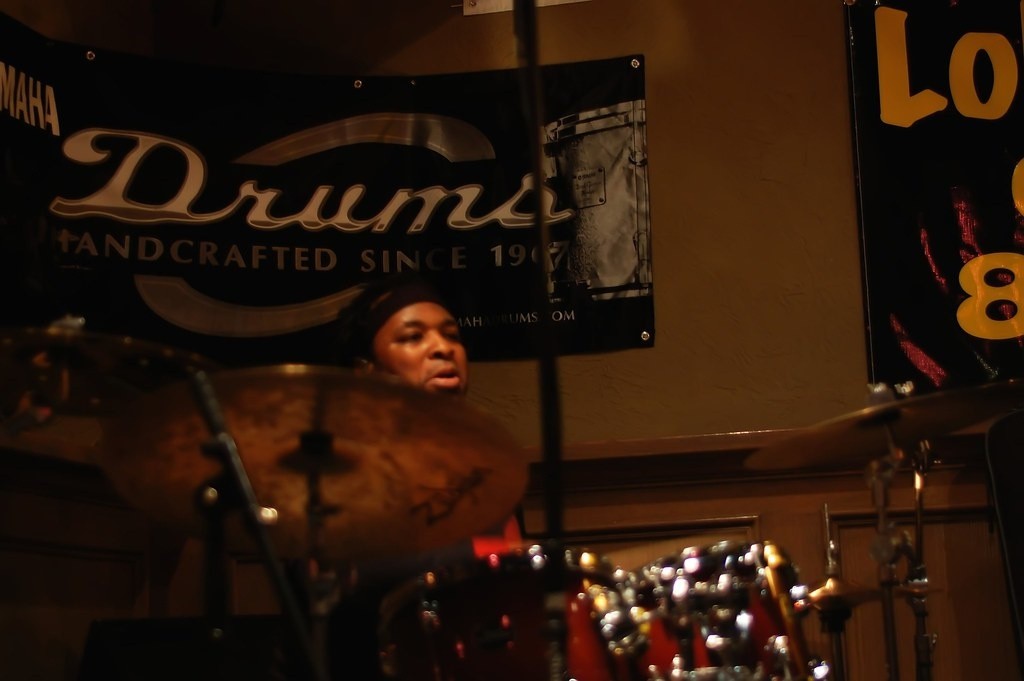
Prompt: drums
<box><xmin>369</xmin><ymin>544</ymin><xmax>653</xmax><ymax>681</ymax></box>
<box><xmin>626</xmin><ymin>538</ymin><xmax>817</xmax><ymax>681</ymax></box>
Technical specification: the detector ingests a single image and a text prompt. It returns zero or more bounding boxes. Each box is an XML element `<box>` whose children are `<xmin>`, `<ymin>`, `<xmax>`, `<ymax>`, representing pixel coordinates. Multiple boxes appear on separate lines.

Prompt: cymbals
<box><xmin>0</xmin><ymin>326</ymin><xmax>227</xmax><ymax>463</ymax></box>
<box><xmin>91</xmin><ymin>361</ymin><xmax>529</xmax><ymax>562</ymax></box>
<box><xmin>742</xmin><ymin>374</ymin><xmax>1024</xmax><ymax>468</ymax></box>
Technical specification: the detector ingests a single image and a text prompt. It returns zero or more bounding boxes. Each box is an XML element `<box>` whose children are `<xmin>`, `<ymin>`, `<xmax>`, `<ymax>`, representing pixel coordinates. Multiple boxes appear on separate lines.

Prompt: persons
<box><xmin>327</xmin><ymin>286</ymin><xmax>473</xmax><ymax>410</ymax></box>
